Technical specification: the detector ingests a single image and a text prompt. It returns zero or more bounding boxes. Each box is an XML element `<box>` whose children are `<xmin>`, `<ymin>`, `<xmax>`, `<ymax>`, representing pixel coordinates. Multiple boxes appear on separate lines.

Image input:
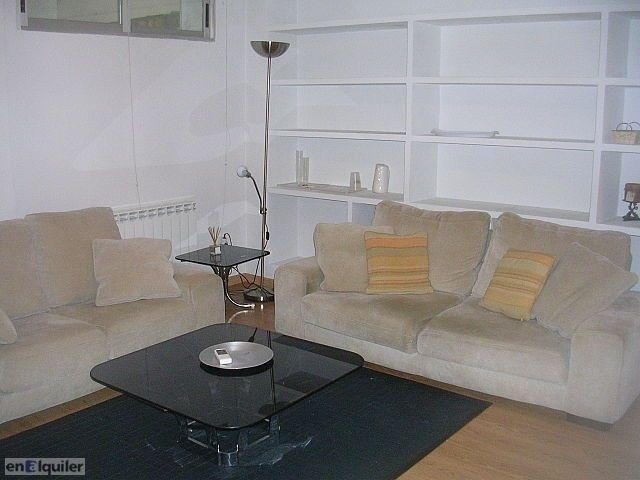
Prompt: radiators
<box><xmin>109</xmin><ymin>201</ymin><xmax>198</xmax><ymax>260</ymax></box>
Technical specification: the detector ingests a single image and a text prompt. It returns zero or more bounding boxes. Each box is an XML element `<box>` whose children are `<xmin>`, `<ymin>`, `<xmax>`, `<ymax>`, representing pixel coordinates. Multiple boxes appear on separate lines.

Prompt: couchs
<box><xmin>0</xmin><ymin>206</ymin><xmax>226</xmax><ymax>427</ymax></box>
<box><xmin>274</xmin><ymin>198</ymin><xmax>639</xmax><ymax>430</ymax></box>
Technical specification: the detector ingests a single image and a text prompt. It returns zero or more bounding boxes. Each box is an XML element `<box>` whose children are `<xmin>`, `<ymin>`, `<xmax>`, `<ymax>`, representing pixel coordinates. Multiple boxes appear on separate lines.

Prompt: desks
<box><xmin>177</xmin><ymin>245</ymin><xmax>270</xmax><ymax>301</ymax></box>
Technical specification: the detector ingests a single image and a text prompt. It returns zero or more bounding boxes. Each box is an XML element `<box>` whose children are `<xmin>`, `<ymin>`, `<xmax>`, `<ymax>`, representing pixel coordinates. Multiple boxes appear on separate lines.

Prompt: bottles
<box><xmin>295</xmin><ymin>150</ymin><xmax>309</xmax><ymax>187</ymax></box>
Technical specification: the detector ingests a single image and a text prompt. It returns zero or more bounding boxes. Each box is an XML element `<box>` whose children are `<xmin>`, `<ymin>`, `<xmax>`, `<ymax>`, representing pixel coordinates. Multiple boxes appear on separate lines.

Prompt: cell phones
<box><xmin>214</xmin><ymin>348</ymin><xmax>232</xmax><ymax>364</ymax></box>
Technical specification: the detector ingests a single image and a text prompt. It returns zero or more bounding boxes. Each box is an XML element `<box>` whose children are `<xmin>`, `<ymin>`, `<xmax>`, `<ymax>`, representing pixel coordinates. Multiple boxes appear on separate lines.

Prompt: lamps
<box><xmin>236</xmin><ymin>39</ymin><xmax>290</xmax><ymax>303</ymax></box>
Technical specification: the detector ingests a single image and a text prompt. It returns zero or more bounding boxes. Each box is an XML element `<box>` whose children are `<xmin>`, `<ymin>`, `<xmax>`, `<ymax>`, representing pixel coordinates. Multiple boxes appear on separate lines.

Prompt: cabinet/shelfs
<box><xmin>409</xmin><ymin>3</ymin><xmax>639</xmax><ymax>233</ymax></box>
<box><xmin>265</xmin><ymin>14</ymin><xmax>408</xmax><ymax>204</ymax></box>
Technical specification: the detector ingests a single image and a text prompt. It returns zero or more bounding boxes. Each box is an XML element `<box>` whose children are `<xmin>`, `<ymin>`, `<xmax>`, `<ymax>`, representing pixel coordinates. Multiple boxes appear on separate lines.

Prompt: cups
<box><xmin>372</xmin><ymin>164</ymin><xmax>391</xmax><ymax>194</ymax></box>
<box><xmin>349</xmin><ymin>172</ymin><xmax>361</xmax><ymax>191</ymax></box>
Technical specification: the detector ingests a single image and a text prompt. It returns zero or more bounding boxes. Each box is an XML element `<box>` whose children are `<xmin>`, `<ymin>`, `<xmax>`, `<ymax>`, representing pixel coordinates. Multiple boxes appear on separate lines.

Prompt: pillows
<box><xmin>1</xmin><ymin>306</ymin><xmax>19</xmax><ymax>347</ymax></box>
<box><xmin>471</xmin><ymin>212</ymin><xmax>631</xmax><ymax>299</ymax></box>
<box><xmin>92</xmin><ymin>237</ymin><xmax>182</xmax><ymax>307</ymax></box>
<box><xmin>531</xmin><ymin>242</ymin><xmax>638</xmax><ymax>338</ymax></box>
<box><xmin>23</xmin><ymin>207</ymin><xmax>121</xmax><ymax>308</ymax></box>
<box><xmin>313</xmin><ymin>223</ymin><xmax>394</xmax><ymax>292</ymax></box>
<box><xmin>370</xmin><ymin>199</ymin><xmax>490</xmax><ymax>296</ymax></box>
<box><xmin>362</xmin><ymin>231</ymin><xmax>437</xmax><ymax>294</ymax></box>
<box><xmin>479</xmin><ymin>248</ymin><xmax>555</xmax><ymax>321</ymax></box>
<box><xmin>1</xmin><ymin>220</ymin><xmax>45</xmax><ymax>320</ymax></box>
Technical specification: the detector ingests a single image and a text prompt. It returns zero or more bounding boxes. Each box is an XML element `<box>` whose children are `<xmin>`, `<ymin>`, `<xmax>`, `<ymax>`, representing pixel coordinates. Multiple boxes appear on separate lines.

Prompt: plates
<box><xmin>198</xmin><ymin>341</ymin><xmax>274</xmax><ymax>370</ymax></box>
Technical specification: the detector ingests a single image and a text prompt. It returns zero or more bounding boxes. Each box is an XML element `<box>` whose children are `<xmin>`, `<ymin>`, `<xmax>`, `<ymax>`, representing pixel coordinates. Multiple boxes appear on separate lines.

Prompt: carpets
<box><xmin>1</xmin><ymin>366</ymin><xmax>493</xmax><ymax>478</ymax></box>
